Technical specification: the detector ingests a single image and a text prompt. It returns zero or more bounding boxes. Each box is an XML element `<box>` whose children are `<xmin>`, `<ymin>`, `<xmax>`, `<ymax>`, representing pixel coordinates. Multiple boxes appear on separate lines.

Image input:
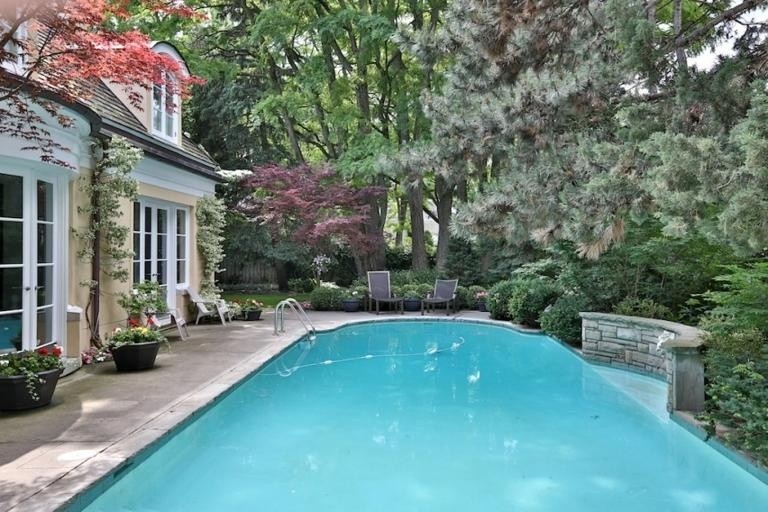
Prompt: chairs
<box><xmin>148</xmin><ymin>308</ymin><xmax>192</xmax><ymax>341</ymax></box>
<box><xmin>421</xmin><ymin>278</ymin><xmax>459</xmax><ymax>315</ymax></box>
<box><xmin>366</xmin><ymin>270</ymin><xmax>404</xmax><ymax>315</ymax></box>
<box><xmin>185</xmin><ymin>287</ymin><xmax>232</xmax><ymax>326</ymax></box>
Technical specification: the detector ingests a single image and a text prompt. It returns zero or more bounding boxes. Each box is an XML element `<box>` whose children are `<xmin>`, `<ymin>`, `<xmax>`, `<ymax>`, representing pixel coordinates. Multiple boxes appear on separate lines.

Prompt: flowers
<box><xmin>338</xmin><ymin>289</ymin><xmax>360</xmax><ymax>301</ymax></box>
<box><xmin>227</xmin><ymin>298</ymin><xmax>265</xmax><ymax>320</ymax></box>
<box><xmin>476</xmin><ymin>291</ymin><xmax>489</xmax><ymax>302</ymax></box>
<box><xmin>0</xmin><ymin>346</ymin><xmax>66</xmax><ymax>403</ymax></box>
<box><xmin>104</xmin><ymin>318</ymin><xmax>171</xmax><ymax>353</ymax></box>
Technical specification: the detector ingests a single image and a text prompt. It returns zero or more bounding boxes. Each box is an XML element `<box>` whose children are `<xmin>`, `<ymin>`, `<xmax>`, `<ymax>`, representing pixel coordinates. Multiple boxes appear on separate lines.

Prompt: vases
<box><xmin>108</xmin><ymin>341</ymin><xmax>162</xmax><ymax>372</ymax></box>
<box><xmin>232</xmin><ymin>310</ymin><xmax>263</xmax><ymax>321</ymax></box>
<box><xmin>0</xmin><ymin>369</ymin><xmax>60</xmax><ymax>411</ymax></box>
<box><xmin>478</xmin><ymin>302</ymin><xmax>488</xmax><ymax>312</ymax></box>
<box><xmin>341</xmin><ymin>301</ymin><xmax>361</xmax><ymax>312</ymax></box>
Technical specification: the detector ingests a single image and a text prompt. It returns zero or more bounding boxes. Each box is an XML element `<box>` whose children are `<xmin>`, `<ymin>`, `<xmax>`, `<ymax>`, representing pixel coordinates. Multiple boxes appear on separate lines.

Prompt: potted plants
<box><xmin>403</xmin><ymin>290</ymin><xmax>423</xmax><ymax>311</ymax></box>
